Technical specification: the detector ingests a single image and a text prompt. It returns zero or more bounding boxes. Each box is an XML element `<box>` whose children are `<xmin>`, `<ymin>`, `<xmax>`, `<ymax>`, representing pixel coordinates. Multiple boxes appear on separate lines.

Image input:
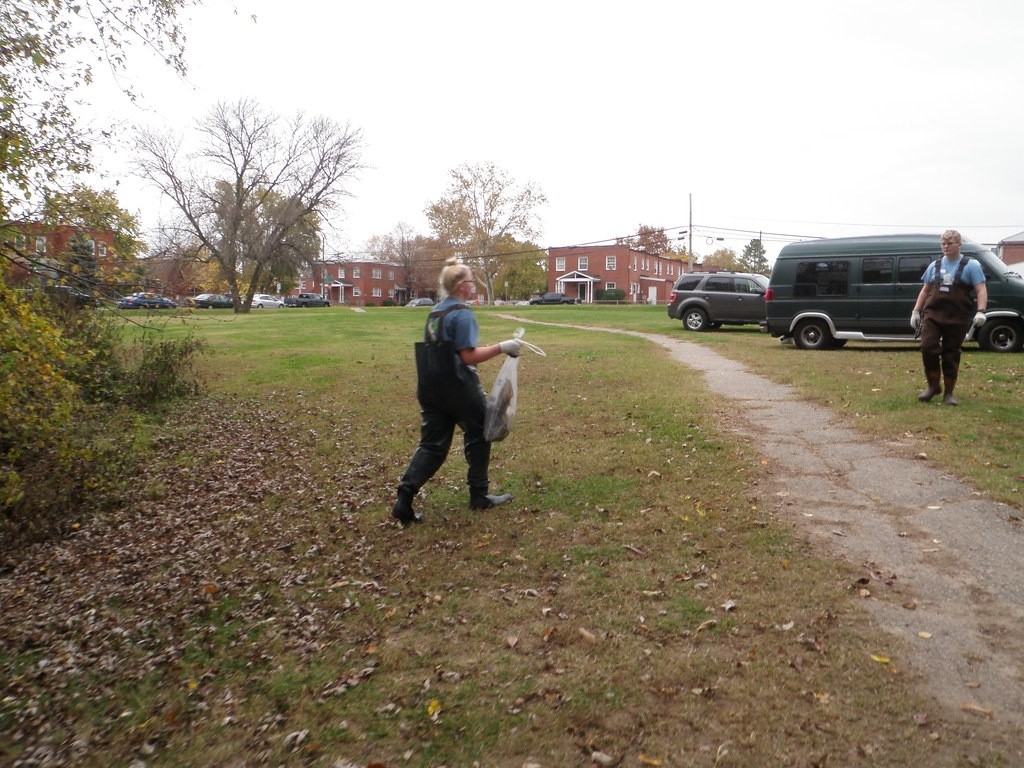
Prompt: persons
<box><xmin>391</xmin><ymin>255</ymin><xmax>522</xmax><ymax>523</ymax></box>
<box><xmin>910</xmin><ymin>229</ymin><xmax>988</xmax><ymax>407</ymax></box>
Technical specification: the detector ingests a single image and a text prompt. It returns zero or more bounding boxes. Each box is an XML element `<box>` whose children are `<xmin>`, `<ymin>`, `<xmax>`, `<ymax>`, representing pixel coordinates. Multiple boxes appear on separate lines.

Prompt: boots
<box><xmin>470</xmin><ymin>482</ymin><xmax>513</xmax><ymax>511</ymax></box>
<box><xmin>943</xmin><ymin>375</ymin><xmax>958</xmax><ymax>406</ymax></box>
<box><xmin>392</xmin><ymin>484</ymin><xmax>424</xmax><ymax>528</ymax></box>
<box><xmin>917</xmin><ymin>368</ymin><xmax>941</xmax><ymax>401</ymax></box>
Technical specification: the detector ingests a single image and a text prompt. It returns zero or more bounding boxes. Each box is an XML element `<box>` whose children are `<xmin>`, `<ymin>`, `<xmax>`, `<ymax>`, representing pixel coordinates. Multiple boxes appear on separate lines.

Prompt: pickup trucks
<box><xmin>284</xmin><ymin>292</ymin><xmax>331</xmax><ymax>308</ymax></box>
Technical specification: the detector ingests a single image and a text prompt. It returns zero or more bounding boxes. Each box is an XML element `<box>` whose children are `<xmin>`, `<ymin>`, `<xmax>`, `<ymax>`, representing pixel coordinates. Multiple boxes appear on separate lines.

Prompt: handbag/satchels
<box><xmin>485</xmin><ymin>328</ymin><xmax>547</xmax><ymax>441</ymax></box>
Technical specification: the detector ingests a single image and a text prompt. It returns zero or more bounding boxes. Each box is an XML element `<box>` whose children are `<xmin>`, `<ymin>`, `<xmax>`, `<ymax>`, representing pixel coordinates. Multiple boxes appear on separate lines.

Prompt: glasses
<box><xmin>941</xmin><ymin>242</ymin><xmax>958</xmax><ymax>245</ymax></box>
<box><xmin>463</xmin><ymin>279</ymin><xmax>476</xmax><ymax>285</ymax></box>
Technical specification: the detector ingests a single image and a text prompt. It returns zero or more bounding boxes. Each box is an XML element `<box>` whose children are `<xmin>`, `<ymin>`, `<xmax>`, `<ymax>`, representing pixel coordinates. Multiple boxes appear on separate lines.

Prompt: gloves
<box><xmin>973</xmin><ymin>312</ymin><xmax>986</xmax><ymax>327</ymax></box>
<box><xmin>910</xmin><ymin>311</ymin><xmax>921</xmax><ymax>329</ymax></box>
<box><xmin>499</xmin><ymin>340</ymin><xmax>521</xmax><ymax>356</ymax></box>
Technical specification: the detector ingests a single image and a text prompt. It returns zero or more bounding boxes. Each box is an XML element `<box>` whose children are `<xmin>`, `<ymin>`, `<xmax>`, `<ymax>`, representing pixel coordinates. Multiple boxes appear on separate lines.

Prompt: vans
<box><xmin>764</xmin><ymin>231</ymin><xmax>1024</xmax><ymax>353</ymax></box>
<box><xmin>667</xmin><ymin>269</ymin><xmax>770</xmax><ymax>332</ymax></box>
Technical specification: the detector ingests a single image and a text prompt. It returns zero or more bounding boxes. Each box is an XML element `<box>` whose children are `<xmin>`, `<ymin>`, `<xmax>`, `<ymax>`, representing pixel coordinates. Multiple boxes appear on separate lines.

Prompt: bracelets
<box><xmin>977</xmin><ymin>309</ymin><xmax>986</xmax><ymax>314</ymax></box>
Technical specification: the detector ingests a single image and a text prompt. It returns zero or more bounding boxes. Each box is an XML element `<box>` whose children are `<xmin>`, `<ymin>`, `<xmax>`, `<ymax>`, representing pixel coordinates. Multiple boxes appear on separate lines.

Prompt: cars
<box><xmin>193</xmin><ymin>293</ymin><xmax>234</xmax><ymax>309</ymax></box>
<box><xmin>529</xmin><ymin>291</ymin><xmax>576</xmax><ymax>305</ymax></box>
<box><xmin>118</xmin><ymin>292</ymin><xmax>177</xmax><ymax>309</ymax></box>
<box><xmin>405</xmin><ymin>297</ymin><xmax>435</xmax><ymax>307</ymax></box>
<box><xmin>241</xmin><ymin>293</ymin><xmax>286</xmax><ymax>309</ymax></box>
